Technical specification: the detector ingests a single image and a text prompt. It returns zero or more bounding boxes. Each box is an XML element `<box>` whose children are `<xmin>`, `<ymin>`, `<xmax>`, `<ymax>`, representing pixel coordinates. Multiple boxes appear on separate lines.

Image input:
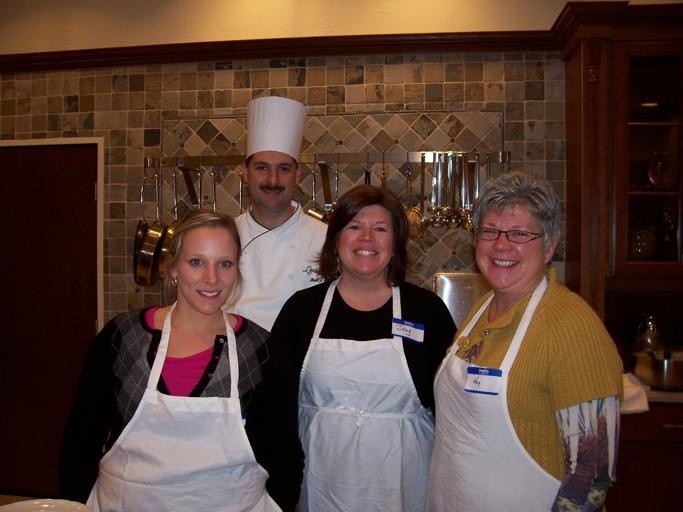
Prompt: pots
<box><xmin>306</xmin><ymin>172</ymin><xmax>326</xmax><ymax>222</ymax></box>
<box><xmin>132</xmin><ymin>170</ymin><xmax>179</xmax><ymax>293</ymax></box>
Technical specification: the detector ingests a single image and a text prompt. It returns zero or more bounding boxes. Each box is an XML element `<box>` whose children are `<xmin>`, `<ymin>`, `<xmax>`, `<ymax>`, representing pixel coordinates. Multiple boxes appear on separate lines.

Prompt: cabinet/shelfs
<box><xmin>564</xmin><ymin>0</ymin><xmax>681</xmax><ymax>510</ymax></box>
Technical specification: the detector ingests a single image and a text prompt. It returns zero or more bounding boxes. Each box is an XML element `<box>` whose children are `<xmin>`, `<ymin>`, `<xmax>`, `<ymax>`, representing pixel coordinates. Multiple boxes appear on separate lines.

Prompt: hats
<box><xmin>246</xmin><ymin>97</ymin><xmax>305</xmax><ymax>163</ymax></box>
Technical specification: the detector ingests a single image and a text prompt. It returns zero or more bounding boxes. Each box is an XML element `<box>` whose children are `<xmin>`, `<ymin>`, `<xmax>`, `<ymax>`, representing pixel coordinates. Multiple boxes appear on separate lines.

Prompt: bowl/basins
<box><xmin>632</xmin><ymin>349</ymin><xmax>683</xmax><ymax>390</ymax></box>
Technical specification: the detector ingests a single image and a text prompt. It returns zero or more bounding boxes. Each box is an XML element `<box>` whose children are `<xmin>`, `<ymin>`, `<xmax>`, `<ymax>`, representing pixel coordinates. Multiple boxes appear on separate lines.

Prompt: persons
<box><xmin>270</xmin><ymin>184</ymin><xmax>458</xmax><ymax>512</ymax></box>
<box><xmin>426</xmin><ymin>169</ymin><xmax>623</xmax><ymax>512</ymax></box>
<box><xmin>220</xmin><ymin>96</ymin><xmax>338</xmax><ymax>332</ymax></box>
<box><xmin>57</xmin><ymin>209</ymin><xmax>305</xmax><ymax>512</ymax></box>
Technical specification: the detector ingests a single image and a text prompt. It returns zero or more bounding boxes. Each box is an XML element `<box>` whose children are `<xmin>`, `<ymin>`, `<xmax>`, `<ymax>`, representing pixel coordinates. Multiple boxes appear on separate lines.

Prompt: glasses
<box><xmin>476</xmin><ymin>227</ymin><xmax>543</xmax><ymax>244</ymax></box>
